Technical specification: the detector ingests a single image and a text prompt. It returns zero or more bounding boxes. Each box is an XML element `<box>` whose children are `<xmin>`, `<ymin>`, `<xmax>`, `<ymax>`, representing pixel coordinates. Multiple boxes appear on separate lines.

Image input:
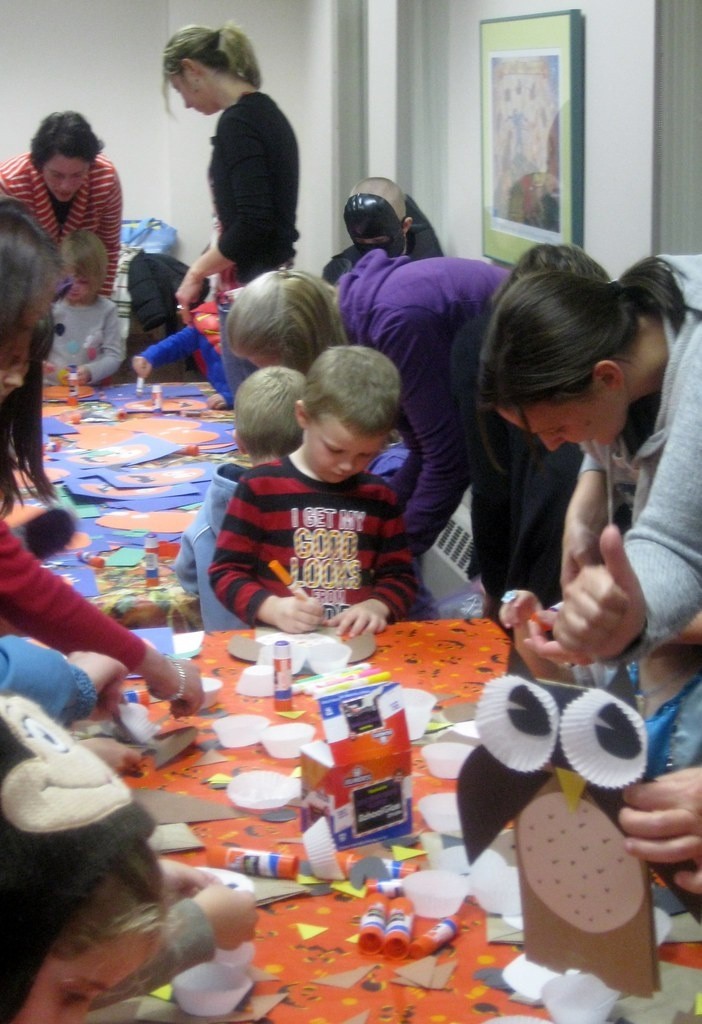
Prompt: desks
<box><xmin>72</xmin><ymin>619</ymin><xmax>702</xmax><ymax>1024</ymax></box>
<box><xmin>0</xmin><ymin>380</ymin><xmax>254</xmax><ymax>631</ymax></box>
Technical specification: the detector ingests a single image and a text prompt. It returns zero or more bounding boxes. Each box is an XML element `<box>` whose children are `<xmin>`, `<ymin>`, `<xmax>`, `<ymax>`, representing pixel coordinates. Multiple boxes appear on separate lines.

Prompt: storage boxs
<box><xmin>297</xmin><ymin>670</ymin><xmax>411</xmax><ymax>853</ymax></box>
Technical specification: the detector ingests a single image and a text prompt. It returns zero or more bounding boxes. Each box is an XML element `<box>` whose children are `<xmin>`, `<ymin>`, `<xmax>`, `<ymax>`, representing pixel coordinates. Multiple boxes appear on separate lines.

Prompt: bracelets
<box><xmin>162</xmin><ymin>653</ymin><xmax>187</xmax><ymax>700</ymax></box>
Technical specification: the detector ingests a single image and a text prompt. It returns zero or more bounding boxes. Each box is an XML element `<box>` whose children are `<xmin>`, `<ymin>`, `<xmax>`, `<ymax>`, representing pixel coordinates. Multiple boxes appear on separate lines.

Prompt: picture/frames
<box><xmin>479</xmin><ymin>5</ymin><xmax>586</xmax><ymax>267</ymax></box>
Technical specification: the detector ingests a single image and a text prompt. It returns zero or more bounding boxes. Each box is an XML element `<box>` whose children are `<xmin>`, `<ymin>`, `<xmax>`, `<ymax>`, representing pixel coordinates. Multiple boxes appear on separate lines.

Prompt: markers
<box><xmin>136</xmin><ymin>357</ymin><xmax>145</xmax><ymax>397</ymax></box>
<box><xmin>268</xmin><ymin>560</ymin><xmax>328</xmax><ymax>628</ymax></box>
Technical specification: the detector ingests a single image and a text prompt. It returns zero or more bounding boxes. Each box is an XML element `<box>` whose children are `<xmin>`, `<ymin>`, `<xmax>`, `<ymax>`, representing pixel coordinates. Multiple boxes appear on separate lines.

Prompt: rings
<box><xmin>177</xmin><ymin>303</ymin><xmax>185</xmax><ymax>311</ymax></box>
<box><xmin>500</xmin><ymin>589</ymin><xmax>519</xmax><ymax>604</ymax></box>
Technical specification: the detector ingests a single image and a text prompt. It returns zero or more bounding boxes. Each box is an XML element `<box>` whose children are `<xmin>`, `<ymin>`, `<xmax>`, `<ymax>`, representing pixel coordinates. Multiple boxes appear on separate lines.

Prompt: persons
<box><xmin>161</xmin><ymin>24</ymin><xmax>301</xmax><ymax>323</ymax></box>
<box><xmin>0</xmin><ymin>109</ymin><xmax>702</xmax><ymax>1024</ymax></box>
